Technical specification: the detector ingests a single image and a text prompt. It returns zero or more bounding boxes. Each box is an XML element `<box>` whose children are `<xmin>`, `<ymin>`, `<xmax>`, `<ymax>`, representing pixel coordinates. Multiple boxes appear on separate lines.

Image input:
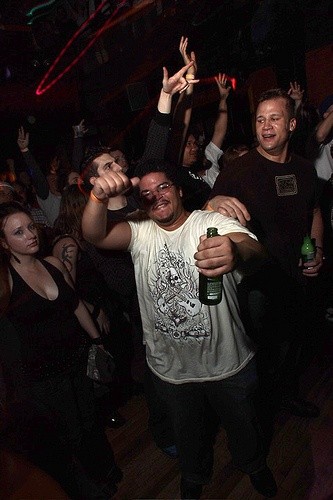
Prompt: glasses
<box><xmin>143</xmin><ymin>182</ymin><xmax>174</xmax><ymax>201</ymax></box>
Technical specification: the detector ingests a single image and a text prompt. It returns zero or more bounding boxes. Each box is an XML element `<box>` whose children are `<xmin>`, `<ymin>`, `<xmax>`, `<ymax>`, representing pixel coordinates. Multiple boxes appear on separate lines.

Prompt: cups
<box><xmin>75</xmin><ymin>126</ymin><xmax>83</xmax><ymax>136</ymax></box>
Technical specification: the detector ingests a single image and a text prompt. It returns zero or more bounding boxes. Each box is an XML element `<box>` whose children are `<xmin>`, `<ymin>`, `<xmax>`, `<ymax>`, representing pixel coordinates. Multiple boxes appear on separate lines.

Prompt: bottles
<box><xmin>301</xmin><ymin>236</ymin><xmax>314</xmax><ymax>270</ymax></box>
<box><xmin>199</xmin><ymin>228</ymin><xmax>223</xmax><ymax>305</ymax></box>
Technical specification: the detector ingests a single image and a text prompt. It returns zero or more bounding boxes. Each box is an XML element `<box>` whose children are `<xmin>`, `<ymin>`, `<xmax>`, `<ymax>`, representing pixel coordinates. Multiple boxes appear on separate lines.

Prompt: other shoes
<box><xmin>181</xmin><ymin>477</ymin><xmax>203</xmax><ymax>500</ymax></box>
<box><xmin>249</xmin><ymin>466</ymin><xmax>277</xmax><ymax>498</ymax></box>
<box><xmin>325</xmin><ymin>308</ymin><xmax>333</xmax><ymax>323</ymax></box>
<box><xmin>281</xmin><ymin>398</ymin><xmax>320</xmax><ymax>418</ymax></box>
<box><xmin>96</xmin><ymin>462</ymin><xmax>123</xmax><ymax>500</ymax></box>
<box><xmin>119</xmin><ymin>382</ymin><xmax>149</xmax><ymax>398</ymax></box>
<box><xmin>106</xmin><ymin>415</ymin><xmax>122</xmax><ymax>428</ymax></box>
<box><xmin>161</xmin><ymin>443</ymin><xmax>180</xmax><ymax>459</ymax></box>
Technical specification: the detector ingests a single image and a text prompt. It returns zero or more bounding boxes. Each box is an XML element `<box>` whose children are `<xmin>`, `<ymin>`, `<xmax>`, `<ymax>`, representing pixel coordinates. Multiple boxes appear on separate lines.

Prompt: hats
<box><xmin>0</xmin><ymin>181</ymin><xmax>16</xmax><ymax>191</ymax></box>
<box><xmin>319</xmin><ymin>95</ymin><xmax>333</xmax><ymax>120</ymax></box>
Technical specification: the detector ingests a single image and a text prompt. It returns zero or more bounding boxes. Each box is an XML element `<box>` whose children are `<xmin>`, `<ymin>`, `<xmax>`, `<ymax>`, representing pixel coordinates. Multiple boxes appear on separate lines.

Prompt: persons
<box><xmin>82</xmin><ymin>167</ymin><xmax>278</xmax><ymax>500</ymax></box>
<box><xmin>0</xmin><ymin>34</ymin><xmax>333</xmax><ymax>458</ymax></box>
<box><xmin>0</xmin><ymin>202</ymin><xmax>125</xmax><ymax>499</ymax></box>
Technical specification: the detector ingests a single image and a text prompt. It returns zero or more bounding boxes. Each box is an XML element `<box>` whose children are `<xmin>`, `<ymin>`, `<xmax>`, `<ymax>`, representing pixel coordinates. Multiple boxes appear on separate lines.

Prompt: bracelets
<box><xmin>89</xmin><ymin>336</ymin><xmax>105</xmax><ymax>346</ymax></box>
<box><xmin>314</xmin><ymin>246</ymin><xmax>323</xmax><ymax>251</ymax></box>
<box><xmin>217</xmin><ymin>109</ymin><xmax>227</xmax><ymax>113</ymax></box>
<box><xmin>91</xmin><ymin>304</ymin><xmax>101</xmax><ymax>321</ymax></box>
